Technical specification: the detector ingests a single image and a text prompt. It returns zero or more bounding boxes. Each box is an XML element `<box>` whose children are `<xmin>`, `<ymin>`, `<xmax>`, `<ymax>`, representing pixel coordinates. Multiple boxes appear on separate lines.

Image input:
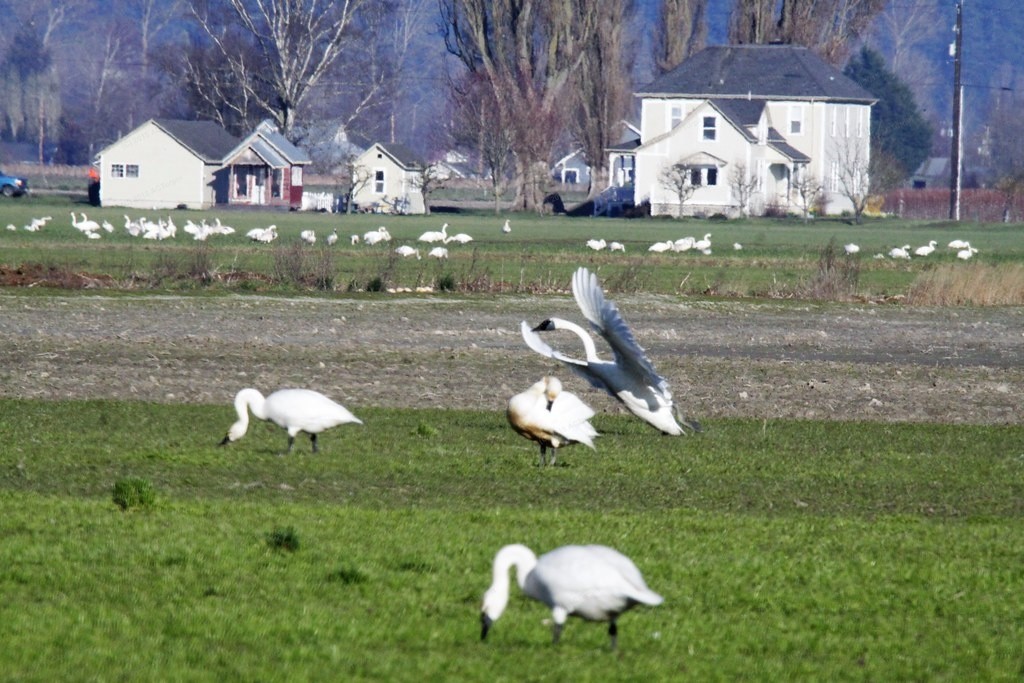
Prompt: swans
<box><xmin>218</xmin><ymin>387</ymin><xmax>364</xmax><ymax>454</ymax></box>
<box><xmin>2</xmin><ymin>209</ymin><xmax>980</xmax><ymax>260</ymax></box>
<box><xmin>521</xmin><ymin>264</ymin><xmax>698</xmax><ymax>438</ymax></box>
<box><xmin>505</xmin><ymin>375</ymin><xmax>601</xmax><ymax>468</ymax></box>
<box><xmin>478</xmin><ymin>544</ymin><xmax>662</xmax><ymax>651</ymax></box>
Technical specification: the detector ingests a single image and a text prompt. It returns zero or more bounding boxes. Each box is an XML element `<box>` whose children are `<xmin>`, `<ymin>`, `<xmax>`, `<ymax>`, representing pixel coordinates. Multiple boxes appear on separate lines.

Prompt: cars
<box><xmin>0</xmin><ymin>170</ymin><xmax>27</xmax><ymax>199</ymax></box>
